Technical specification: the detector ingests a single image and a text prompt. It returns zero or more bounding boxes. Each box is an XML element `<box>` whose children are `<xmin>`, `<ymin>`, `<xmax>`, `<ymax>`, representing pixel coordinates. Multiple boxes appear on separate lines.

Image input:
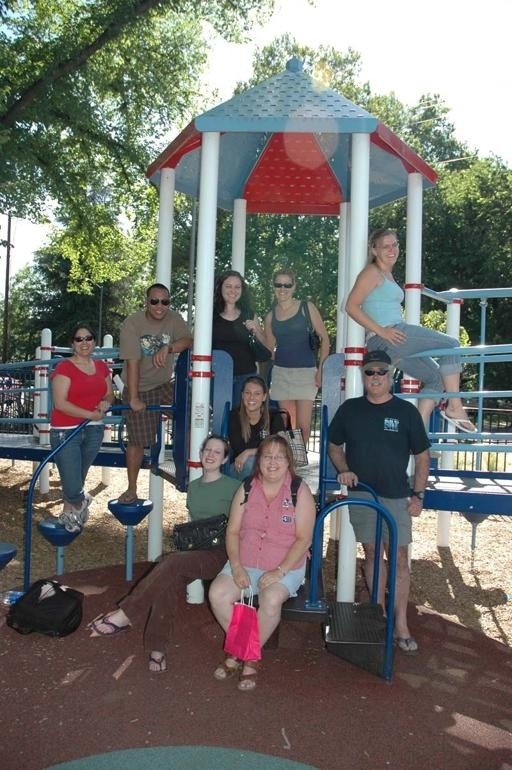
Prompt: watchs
<box><xmin>412</xmin><ymin>489</ymin><xmax>425</xmax><ymax>500</ymax></box>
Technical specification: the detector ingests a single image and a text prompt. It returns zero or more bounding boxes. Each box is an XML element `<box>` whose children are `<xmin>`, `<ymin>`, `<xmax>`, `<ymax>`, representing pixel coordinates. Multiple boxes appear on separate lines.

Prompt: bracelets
<box><xmin>166</xmin><ymin>342</ymin><xmax>174</xmax><ymax>355</ymax></box>
<box><xmin>276</xmin><ymin>564</ymin><xmax>287</xmax><ymax>577</ymax></box>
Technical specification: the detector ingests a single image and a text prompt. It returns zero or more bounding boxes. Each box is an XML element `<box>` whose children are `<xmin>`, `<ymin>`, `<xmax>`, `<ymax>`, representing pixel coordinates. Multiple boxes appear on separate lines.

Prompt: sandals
<box><xmin>237</xmin><ymin>660</ymin><xmax>260</xmax><ymax>691</ymax></box>
<box><xmin>213</xmin><ymin>655</ymin><xmax>240</xmax><ymax>681</ymax></box>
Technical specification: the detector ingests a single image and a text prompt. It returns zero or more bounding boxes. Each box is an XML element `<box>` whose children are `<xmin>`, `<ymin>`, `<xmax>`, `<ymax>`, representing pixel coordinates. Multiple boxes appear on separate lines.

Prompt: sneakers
<box><xmin>58</xmin><ymin>514</ymin><xmax>81</xmax><ymax>534</ymax></box>
<box><xmin>71</xmin><ymin>492</ymin><xmax>91</xmax><ymax>523</ymax></box>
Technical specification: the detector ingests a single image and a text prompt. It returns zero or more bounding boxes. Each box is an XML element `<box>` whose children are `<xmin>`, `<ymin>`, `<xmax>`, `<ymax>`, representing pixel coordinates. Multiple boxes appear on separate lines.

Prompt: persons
<box><xmin>89</xmin><ymin>434</ymin><xmax>247</xmax><ymax>675</ymax></box>
<box><xmin>117</xmin><ymin>283</ymin><xmax>193</xmax><ymax>505</ymax></box>
<box><xmin>342</xmin><ymin>226</ymin><xmax>479</xmax><ymax>435</ymax></box>
<box><xmin>242</xmin><ymin>268</ymin><xmax>330</xmax><ymax>446</ymax></box>
<box><xmin>324</xmin><ymin>350</ymin><xmax>431</xmax><ymax>656</ymax></box>
<box><xmin>209</xmin><ymin>435</ymin><xmax>317</xmax><ymax>694</ymax></box>
<box><xmin>218</xmin><ymin>377</ymin><xmax>286</xmax><ymax>479</ymax></box>
<box><xmin>212</xmin><ymin>269</ymin><xmax>259</xmax><ymax>409</ymax></box>
<box><xmin>47</xmin><ymin>325</ymin><xmax>117</xmax><ymax>534</ymax></box>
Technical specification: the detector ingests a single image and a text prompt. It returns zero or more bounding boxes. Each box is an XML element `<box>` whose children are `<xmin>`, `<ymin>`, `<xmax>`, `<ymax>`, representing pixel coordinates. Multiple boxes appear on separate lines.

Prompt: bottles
<box><xmin>0</xmin><ymin>591</ymin><xmax>27</xmax><ymax>606</ymax></box>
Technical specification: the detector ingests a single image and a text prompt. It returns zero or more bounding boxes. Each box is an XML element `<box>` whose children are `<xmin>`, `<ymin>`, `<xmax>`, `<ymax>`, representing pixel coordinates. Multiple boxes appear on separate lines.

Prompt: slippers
<box><xmin>148</xmin><ymin>653</ymin><xmax>168</xmax><ymax>674</ymax></box>
<box><xmin>440</xmin><ymin>410</ymin><xmax>478</xmax><ymax>433</ymax></box>
<box><xmin>393</xmin><ymin>631</ymin><xmax>420</xmax><ymax>656</ymax></box>
<box><xmin>93</xmin><ymin>610</ymin><xmax>130</xmax><ymax>636</ymax></box>
<box><xmin>118</xmin><ymin>491</ymin><xmax>137</xmax><ymax>505</ymax></box>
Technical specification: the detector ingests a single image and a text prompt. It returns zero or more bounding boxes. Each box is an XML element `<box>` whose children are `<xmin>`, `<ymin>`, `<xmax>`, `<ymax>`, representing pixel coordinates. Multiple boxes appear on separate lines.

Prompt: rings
<box><xmin>238</xmin><ymin>463</ymin><xmax>241</xmax><ymax>468</ymax></box>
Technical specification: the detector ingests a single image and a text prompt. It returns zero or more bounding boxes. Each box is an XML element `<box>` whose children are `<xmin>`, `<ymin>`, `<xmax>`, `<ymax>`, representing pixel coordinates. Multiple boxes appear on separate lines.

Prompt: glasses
<box><xmin>147</xmin><ymin>298</ymin><xmax>170</xmax><ymax>306</ymax></box>
<box><xmin>364</xmin><ymin>368</ymin><xmax>388</xmax><ymax>377</ymax></box>
<box><xmin>373</xmin><ymin>241</ymin><xmax>399</xmax><ymax>249</ymax></box>
<box><xmin>273</xmin><ymin>281</ymin><xmax>293</xmax><ymax>288</ymax></box>
<box><xmin>73</xmin><ymin>335</ymin><xmax>93</xmax><ymax>342</ymax></box>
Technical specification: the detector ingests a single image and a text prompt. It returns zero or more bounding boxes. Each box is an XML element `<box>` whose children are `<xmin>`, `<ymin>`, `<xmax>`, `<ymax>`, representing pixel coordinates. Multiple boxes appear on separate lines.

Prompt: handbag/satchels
<box><xmin>173</xmin><ymin>514</ymin><xmax>228</xmax><ymax>551</ymax></box>
<box><xmin>6</xmin><ymin>579</ymin><xmax>84</xmax><ymax>638</ymax></box>
<box><xmin>308</xmin><ymin>328</ymin><xmax>321</xmax><ymax>351</ymax></box>
<box><xmin>277</xmin><ymin>429</ymin><xmax>307</xmax><ymax>467</ymax></box>
<box><xmin>248</xmin><ymin>334</ymin><xmax>272</xmax><ymax>362</ymax></box>
<box><xmin>223</xmin><ymin>586</ymin><xmax>262</xmax><ymax>662</ymax></box>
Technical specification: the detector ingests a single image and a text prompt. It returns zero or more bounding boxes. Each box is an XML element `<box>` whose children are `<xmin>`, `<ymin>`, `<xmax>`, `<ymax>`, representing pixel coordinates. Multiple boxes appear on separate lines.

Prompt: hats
<box><xmin>362</xmin><ymin>350</ymin><xmax>391</xmax><ymax>367</ymax></box>
